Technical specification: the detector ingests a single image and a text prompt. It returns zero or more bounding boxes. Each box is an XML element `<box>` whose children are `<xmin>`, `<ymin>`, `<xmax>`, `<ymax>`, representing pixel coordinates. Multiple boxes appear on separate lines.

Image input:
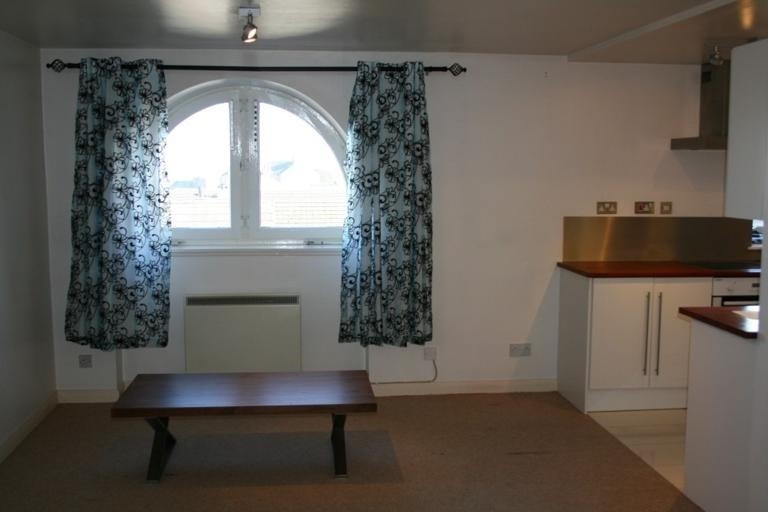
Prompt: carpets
<box><xmin>0</xmin><ymin>392</ymin><xmax>705</xmax><ymax>512</ymax></box>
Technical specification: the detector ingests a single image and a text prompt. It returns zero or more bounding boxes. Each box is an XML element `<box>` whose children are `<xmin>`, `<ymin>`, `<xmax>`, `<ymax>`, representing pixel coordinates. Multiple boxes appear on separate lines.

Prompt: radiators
<box><xmin>185</xmin><ymin>293</ymin><xmax>302</xmax><ymax>374</ymax></box>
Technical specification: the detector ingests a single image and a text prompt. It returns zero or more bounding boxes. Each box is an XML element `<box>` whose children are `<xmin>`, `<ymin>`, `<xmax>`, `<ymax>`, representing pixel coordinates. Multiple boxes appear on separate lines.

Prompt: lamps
<box><xmin>239</xmin><ymin>7</ymin><xmax>261</xmax><ymax>43</ymax></box>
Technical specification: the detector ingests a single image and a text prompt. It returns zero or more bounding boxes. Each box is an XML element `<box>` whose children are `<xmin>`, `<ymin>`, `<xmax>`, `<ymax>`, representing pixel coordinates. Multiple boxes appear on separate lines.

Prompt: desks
<box><xmin>111</xmin><ymin>370</ymin><xmax>377</xmax><ymax>481</ymax></box>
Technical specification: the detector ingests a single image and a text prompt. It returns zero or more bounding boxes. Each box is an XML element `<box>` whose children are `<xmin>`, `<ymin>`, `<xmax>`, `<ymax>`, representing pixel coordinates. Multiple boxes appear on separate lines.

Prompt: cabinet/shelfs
<box><xmin>714</xmin><ymin>275</ymin><xmax>761</xmax><ymax>307</ymax></box>
<box><xmin>589</xmin><ymin>276</ymin><xmax>711</xmax><ymax>391</ymax></box>
<box><xmin>669</xmin><ymin>60</ymin><xmax>734</xmax><ymax>152</ymax></box>
